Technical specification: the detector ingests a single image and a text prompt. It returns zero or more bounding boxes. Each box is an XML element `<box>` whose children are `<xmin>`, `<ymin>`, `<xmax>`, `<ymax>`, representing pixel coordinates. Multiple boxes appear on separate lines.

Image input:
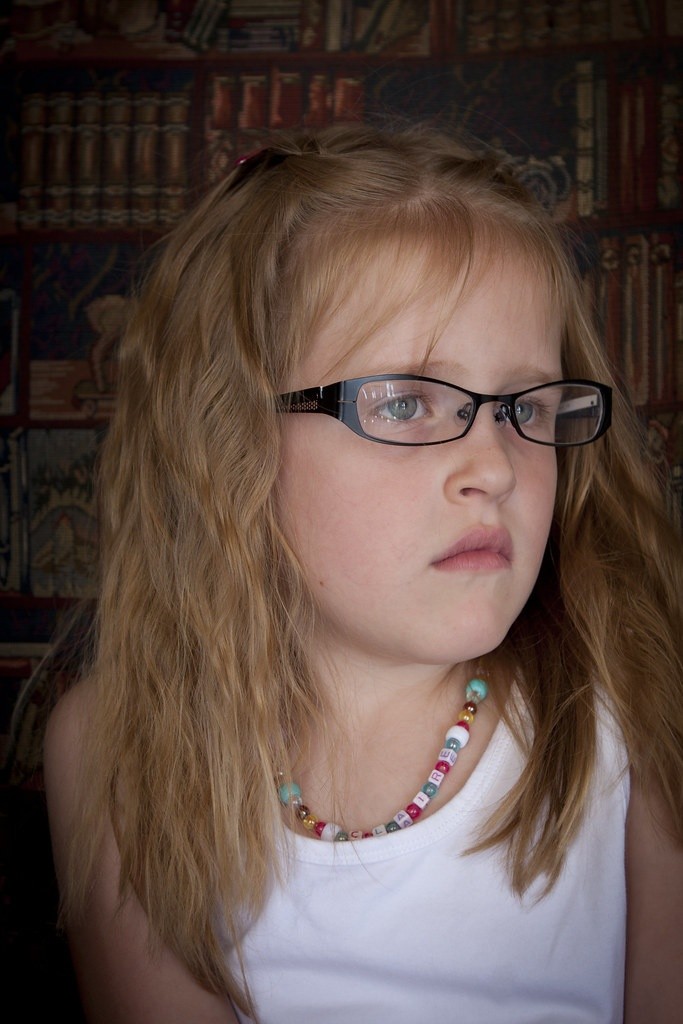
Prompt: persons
<box><xmin>44</xmin><ymin>103</ymin><xmax>682</xmax><ymax>1024</ymax></box>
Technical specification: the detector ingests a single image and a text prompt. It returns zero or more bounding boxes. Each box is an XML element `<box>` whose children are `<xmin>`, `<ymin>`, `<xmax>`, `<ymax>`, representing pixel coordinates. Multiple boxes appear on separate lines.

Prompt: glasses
<box><xmin>276</xmin><ymin>375</ymin><xmax>614</xmax><ymax>446</ymax></box>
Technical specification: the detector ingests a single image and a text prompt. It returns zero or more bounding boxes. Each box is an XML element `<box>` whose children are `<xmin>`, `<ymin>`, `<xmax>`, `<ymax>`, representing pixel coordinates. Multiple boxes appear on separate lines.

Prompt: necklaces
<box><xmin>272</xmin><ymin>670</ymin><xmax>491</xmax><ymax>840</ymax></box>
